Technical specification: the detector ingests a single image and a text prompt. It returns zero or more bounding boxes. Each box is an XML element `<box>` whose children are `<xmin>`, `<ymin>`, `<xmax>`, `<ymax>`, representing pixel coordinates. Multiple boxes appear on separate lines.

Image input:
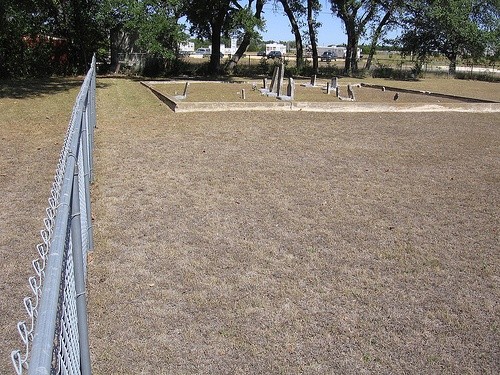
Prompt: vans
<box><xmin>195</xmin><ymin>48</ymin><xmax>210</xmax><ymax>55</ymax></box>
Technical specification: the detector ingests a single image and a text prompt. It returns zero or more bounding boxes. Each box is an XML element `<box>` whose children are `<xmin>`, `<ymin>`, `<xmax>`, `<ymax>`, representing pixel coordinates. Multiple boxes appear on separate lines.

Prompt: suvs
<box><xmin>321</xmin><ymin>50</ymin><xmax>337</xmax><ymax>62</ymax></box>
<box><xmin>267</xmin><ymin>51</ymin><xmax>281</xmax><ymax>60</ymax></box>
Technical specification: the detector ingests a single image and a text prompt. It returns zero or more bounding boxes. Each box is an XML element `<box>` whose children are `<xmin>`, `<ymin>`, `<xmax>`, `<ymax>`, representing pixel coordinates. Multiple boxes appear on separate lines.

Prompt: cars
<box><xmin>220</xmin><ymin>52</ymin><xmax>224</xmax><ymax>58</ymax></box>
<box><xmin>257</xmin><ymin>51</ymin><xmax>265</xmax><ymax>56</ymax></box>
<box><xmin>178</xmin><ymin>51</ymin><xmax>190</xmax><ymax>58</ymax></box>
